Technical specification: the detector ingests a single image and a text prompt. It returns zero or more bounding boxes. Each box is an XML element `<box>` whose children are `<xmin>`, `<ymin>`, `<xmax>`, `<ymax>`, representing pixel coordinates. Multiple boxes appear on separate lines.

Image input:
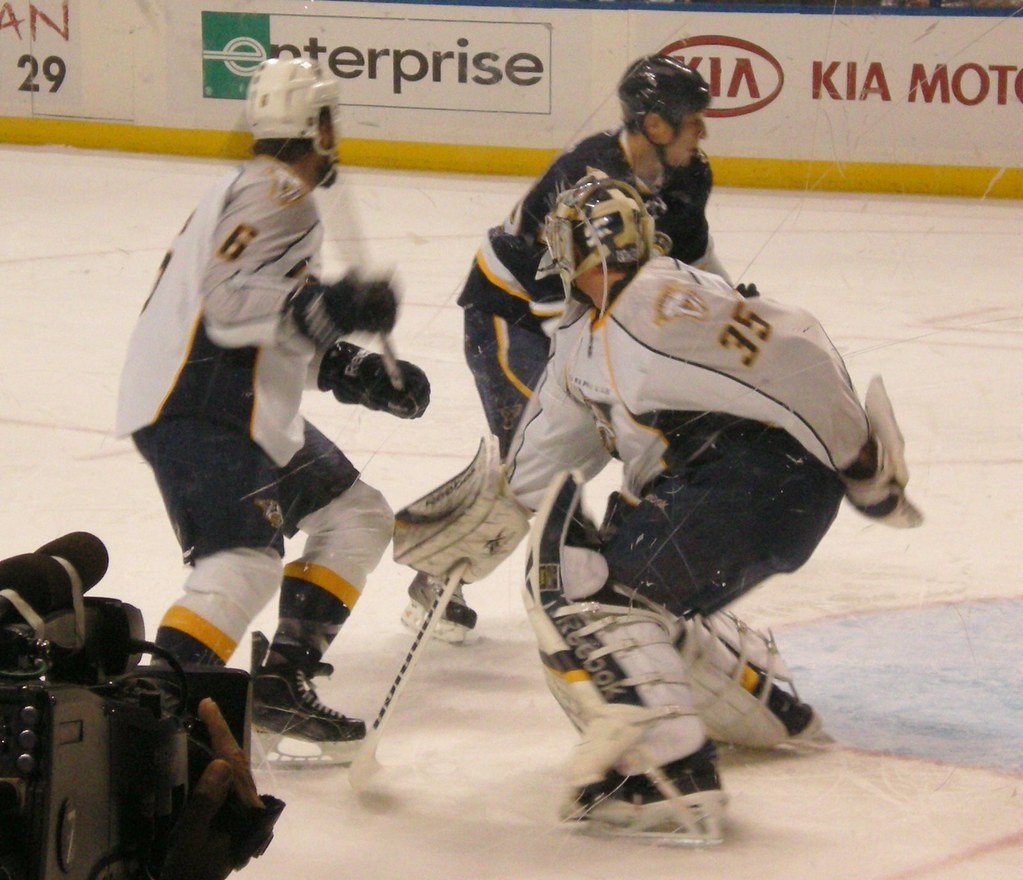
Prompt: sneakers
<box><xmin>558</xmin><ymin>738</ymin><xmax>728</xmax><ymax>846</ymax></box>
<box><xmin>401</xmin><ymin>572</ymin><xmax>477</xmax><ymax>645</ymax></box>
<box><xmin>753</xmin><ymin>676</ymin><xmax>837</xmax><ymax>748</ymax></box>
<box><xmin>249</xmin><ymin>632</ymin><xmax>367</xmax><ymax>770</ymax></box>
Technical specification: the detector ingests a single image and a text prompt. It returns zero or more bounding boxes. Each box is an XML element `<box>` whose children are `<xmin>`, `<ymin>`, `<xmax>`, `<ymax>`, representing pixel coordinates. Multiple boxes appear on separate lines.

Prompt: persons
<box><xmin>135</xmin><ymin>58</ymin><xmax>431</xmax><ymax>744</ymax></box>
<box><xmin>394</xmin><ymin>169</ymin><xmax>921</xmax><ymax>853</ymax></box>
<box><xmin>149</xmin><ymin>696</ymin><xmax>285</xmax><ymax>880</ymax></box>
<box><xmin>458</xmin><ymin>49</ymin><xmax>712</xmax><ymax>461</ymax></box>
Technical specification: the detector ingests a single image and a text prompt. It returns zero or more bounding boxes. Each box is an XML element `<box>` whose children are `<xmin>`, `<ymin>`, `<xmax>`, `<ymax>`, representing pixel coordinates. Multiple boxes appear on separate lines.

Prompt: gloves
<box><xmin>318</xmin><ymin>340</ymin><xmax>432</xmax><ymax>419</ymax></box>
<box><xmin>293</xmin><ymin>270</ymin><xmax>397</xmax><ymax>346</ymax></box>
<box><xmin>735</xmin><ymin>283</ymin><xmax>759</xmax><ymax>298</ymax></box>
<box><xmin>840</xmin><ymin>433</ymin><xmax>897</xmax><ymax>517</ymax></box>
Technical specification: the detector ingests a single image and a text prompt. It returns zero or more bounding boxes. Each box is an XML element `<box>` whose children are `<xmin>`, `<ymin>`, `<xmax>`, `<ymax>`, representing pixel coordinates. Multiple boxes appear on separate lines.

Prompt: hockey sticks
<box><xmin>377</xmin><ymin>332</ymin><xmax>407</xmax><ymax>394</ymax></box>
<box><xmin>346</xmin><ymin>558</ymin><xmax>469</xmax><ymax>787</ymax></box>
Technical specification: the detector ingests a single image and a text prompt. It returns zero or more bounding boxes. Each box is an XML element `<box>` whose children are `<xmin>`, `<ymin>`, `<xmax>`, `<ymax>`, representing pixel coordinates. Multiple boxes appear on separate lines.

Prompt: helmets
<box><xmin>245</xmin><ymin>58</ymin><xmax>340</xmax><ymax>188</ymax></box>
<box><xmin>619</xmin><ymin>54</ymin><xmax>711</xmax><ymax>129</ymax></box>
<box><xmin>534</xmin><ymin>170</ymin><xmax>673</xmax><ymax>329</ymax></box>
